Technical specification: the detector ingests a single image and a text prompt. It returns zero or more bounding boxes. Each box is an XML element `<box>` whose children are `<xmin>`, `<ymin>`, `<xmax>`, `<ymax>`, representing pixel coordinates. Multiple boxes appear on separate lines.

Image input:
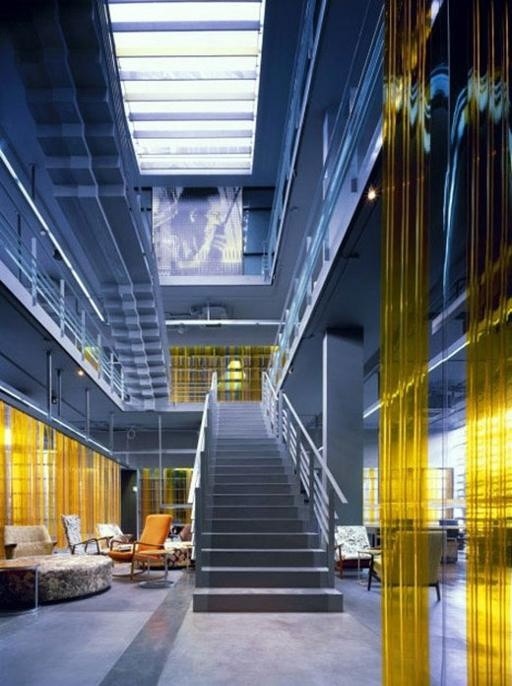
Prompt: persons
<box><xmin>171</xmin><ymin>187</ymin><xmax>227</xmax><ymax>271</ymax></box>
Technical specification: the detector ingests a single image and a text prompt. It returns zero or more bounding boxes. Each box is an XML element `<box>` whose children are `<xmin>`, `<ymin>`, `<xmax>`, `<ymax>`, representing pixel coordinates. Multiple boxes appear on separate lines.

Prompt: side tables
<box><xmin>0</xmin><ymin>559</ymin><xmax>42</xmax><ymax>617</ymax></box>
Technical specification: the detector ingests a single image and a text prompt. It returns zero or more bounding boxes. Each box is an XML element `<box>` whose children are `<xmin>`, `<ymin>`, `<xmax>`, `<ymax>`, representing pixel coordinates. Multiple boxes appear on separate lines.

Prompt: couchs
<box><xmin>0</xmin><ymin>525</ymin><xmax>113</xmax><ymax>603</ymax></box>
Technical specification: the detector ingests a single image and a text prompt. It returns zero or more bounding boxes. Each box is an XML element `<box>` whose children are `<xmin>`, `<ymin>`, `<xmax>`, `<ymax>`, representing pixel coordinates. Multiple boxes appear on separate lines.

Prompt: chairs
<box><xmin>334</xmin><ymin>520</ymin><xmax>460</xmax><ymax>600</ymax></box>
<box><xmin>59</xmin><ymin>515</ymin><xmax>193</xmax><ymax>586</ymax></box>
